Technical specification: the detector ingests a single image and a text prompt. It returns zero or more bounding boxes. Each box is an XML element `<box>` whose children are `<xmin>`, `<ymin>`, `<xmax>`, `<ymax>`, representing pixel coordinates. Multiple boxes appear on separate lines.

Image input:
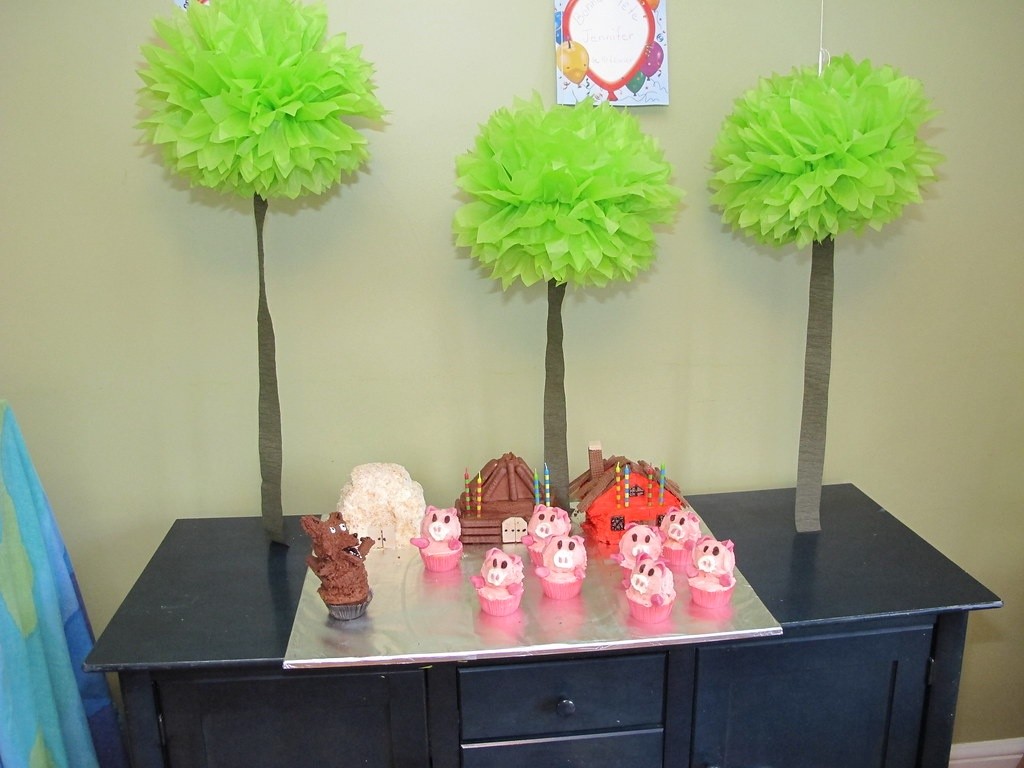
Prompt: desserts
<box><xmin>298</xmin><ymin>511</ymin><xmax>375</xmax><ymax>620</ymax></box>
<box><xmin>410</xmin><ymin>504</ymin><xmax>589</xmax><ymax>615</ymax></box>
<box><xmin>610</xmin><ymin>506</ymin><xmax>737</xmax><ymax>623</ymax></box>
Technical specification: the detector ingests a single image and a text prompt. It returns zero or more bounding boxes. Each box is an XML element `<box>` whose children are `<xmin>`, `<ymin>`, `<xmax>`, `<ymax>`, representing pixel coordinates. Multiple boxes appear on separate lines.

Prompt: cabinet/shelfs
<box><xmin>82</xmin><ymin>482</ymin><xmax>1006</xmax><ymax>768</ymax></box>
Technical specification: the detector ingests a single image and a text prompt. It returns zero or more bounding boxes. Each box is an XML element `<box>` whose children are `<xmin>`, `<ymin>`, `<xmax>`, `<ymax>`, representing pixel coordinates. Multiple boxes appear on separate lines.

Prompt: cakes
<box><xmin>336</xmin><ymin>463</ymin><xmax>425</xmax><ymax>549</ymax></box>
<box><xmin>572</xmin><ymin>444</ymin><xmax>687</xmax><ymax>545</ymax></box>
<box><xmin>453</xmin><ymin>451</ymin><xmax>556</xmax><ymax>545</ymax></box>
